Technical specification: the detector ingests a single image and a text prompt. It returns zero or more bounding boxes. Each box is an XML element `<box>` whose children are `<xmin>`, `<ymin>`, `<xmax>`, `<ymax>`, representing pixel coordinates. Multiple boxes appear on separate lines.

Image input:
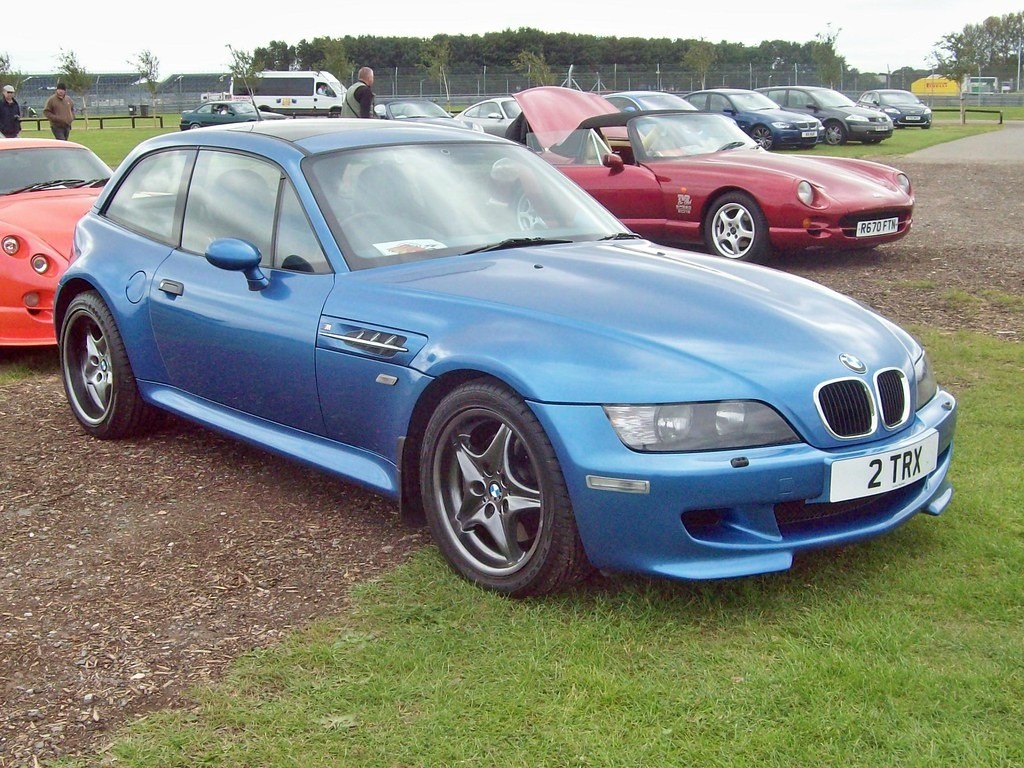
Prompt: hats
<box><xmin>3</xmin><ymin>84</ymin><xmax>15</xmax><ymax>92</ymax></box>
<box><xmin>57</xmin><ymin>83</ymin><xmax>66</xmax><ymax>91</ymax></box>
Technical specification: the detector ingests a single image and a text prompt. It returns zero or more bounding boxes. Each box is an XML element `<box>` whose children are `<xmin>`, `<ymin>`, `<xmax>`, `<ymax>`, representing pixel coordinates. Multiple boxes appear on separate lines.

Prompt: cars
<box><xmin>54</xmin><ymin>118</ymin><xmax>957</xmax><ymax>602</ymax></box>
<box><xmin>680</xmin><ymin>88</ymin><xmax>825</xmax><ymax>151</ymax></box>
<box><xmin>453</xmin><ymin>97</ymin><xmax>523</xmax><ymax>138</ymax></box>
<box><xmin>0</xmin><ymin>138</ymin><xmax>140</xmax><ymax>346</ymax></box>
<box><xmin>753</xmin><ymin>86</ymin><xmax>893</xmax><ymax>146</ymax></box>
<box><xmin>488</xmin><ymin>86</ymin><xmax>915</xmax><ymax>266</ymax></box>
<box><xmin>855</xmin><ymin>89</ymin><xmax>933</xmax><ymax>130</ymax></box>
<box><xmin>371</xmin><ymin>97</ymin><xmax>485</xmax><ymax>133</ymax></box>
<box><xmin>600</xmin><ymin>91</ymin><xmax>738</xmax><ymax>144</ymax></box>
<box><xmin>179</xmin><ymin>100</ymin><xmax>291</xmax><ymax>131</ymax></box>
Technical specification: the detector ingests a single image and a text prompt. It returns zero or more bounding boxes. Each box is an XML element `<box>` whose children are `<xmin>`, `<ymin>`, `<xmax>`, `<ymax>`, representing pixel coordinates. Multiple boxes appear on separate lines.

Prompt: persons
<box><xmin>505</xmin><ymin>111</ymin><xmax>533</xmax><ymax>144</ymax></box>
<box><xmin>0</xmin><ymin>85</ymin><xmax>22</xmax><ymax>138</ymax></box>
<box><xmin>220</xmin><ymin>105</ymin><xmax>228</xmax><ymax>115</ymax></box>
<box><xmin>341</xmin><ymin>66</ymin><xmax>378</xmax><ymax>118</ymax></box>
<box><xmin>22</xmin><ymin>101</ymin><xmax>37</xmax><ymax>117</ymax></box>
<box><xmin>316</xmin><ymin>83</ymin><xmax>328</xmax><ymax>96</ymax></box>
<box><xmin>43</xmin><ymin>83</ymin><xmax>75</xmax><ymax>141</ymax></box>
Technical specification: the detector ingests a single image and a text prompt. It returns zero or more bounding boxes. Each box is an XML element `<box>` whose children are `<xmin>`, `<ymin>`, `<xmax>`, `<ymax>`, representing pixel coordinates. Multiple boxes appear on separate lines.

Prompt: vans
<box><xmin>229</xmin><ymin>71</ymin><xmax>374</xmax><ymax>119</ymax></box>
